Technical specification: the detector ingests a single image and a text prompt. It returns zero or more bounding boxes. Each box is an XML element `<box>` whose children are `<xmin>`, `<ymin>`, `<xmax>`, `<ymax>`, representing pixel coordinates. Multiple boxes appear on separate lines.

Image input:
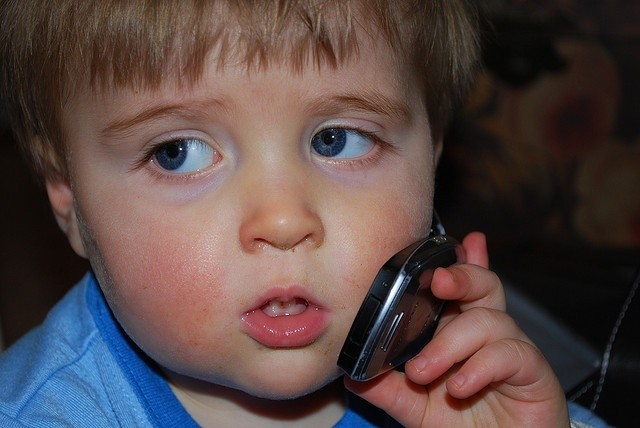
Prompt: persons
<box><xmin>0</xmin><ymin>0</ymin><xmax>615</xmax><ymax>427</ymax></box>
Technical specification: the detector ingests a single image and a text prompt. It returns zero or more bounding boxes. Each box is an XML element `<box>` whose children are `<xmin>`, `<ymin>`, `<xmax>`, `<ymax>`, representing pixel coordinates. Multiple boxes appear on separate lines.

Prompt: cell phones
<box><xmin>336</xmin><ymin>210</ymin><xmax>462</xmax><ymax>381</ymax></box>
